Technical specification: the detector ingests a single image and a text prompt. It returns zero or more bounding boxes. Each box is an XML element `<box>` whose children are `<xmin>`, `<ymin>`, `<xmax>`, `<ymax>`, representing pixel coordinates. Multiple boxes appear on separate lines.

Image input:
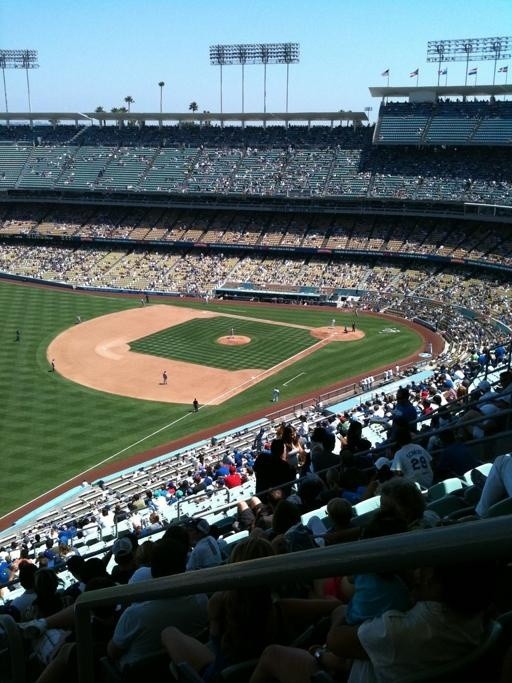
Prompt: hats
<box><xmin>327</xmin><ymin>496</ymin><xmax>351</xmax><ymax>520</ymax></box>
<box><xmin>478</xmin><ymin>380</ymin><xmax>491</xmax><ymax>393</ymax></box>
<box><xmin>111</xmin><ymin>535</ymin><xmax>133</xmax><ymax>557</ymax></box>
<box><xmin>286</xmin><ymin>493</ymin><xmax>305</xmax><ymax>506</ymax></box>
<box><xmin>161</xmin><ymin>449</ymin><xmax>252</xmax><ymax>487</ymax></box>
<box><xmin>188</xmin><ymin>515</ymin><xmax>210</xmax><ymax>534</ymax></box>
<box><xmin>374</xmin><ymin>456</ymin><xmax>390</xmax><ymax>470</ymax></box>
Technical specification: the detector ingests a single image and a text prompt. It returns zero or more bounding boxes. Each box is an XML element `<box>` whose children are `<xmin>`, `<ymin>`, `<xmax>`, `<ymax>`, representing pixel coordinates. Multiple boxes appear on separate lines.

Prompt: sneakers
<box><xmin>16</xmin><ymin>617</ymin><xmax>48</xmax><ymax>639</ymax></box>
<box><xmin>471</xmin><ymin>469</ymin><xmax>490</xmax><ymax>489</ymax></box>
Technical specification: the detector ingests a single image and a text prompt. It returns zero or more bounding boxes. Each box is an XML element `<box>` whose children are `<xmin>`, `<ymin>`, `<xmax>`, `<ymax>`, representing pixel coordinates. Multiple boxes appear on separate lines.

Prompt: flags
<box><xmin>468</xmin><ymin>67</ymin><xmax>478</xmax><ymax>75</ymax></box>
<box><xmin>497</xmin><ymin>66</ymin><xmax>507</xmax><ymax>72</ymax></box>
<box><xmin>438</xmin><ymin>67</ymin><xmax>447</xmax><ymax>75</ymax></box>
<box><xmin>409</xmin><ymin>68</ymin><xmax>418</xmax><ymax>78</ymax></box>
<box><xmin>381</xmin><ymin>67</ymin><xmax>389</xmax><ymax>77</ymax></box>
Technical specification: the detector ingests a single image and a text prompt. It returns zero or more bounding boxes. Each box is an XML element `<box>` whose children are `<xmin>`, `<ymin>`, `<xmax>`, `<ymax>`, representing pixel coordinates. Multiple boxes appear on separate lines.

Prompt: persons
<box><xmin>76</xmin><ymin>314</ymin><xmax>83</xmax><ymax>324</ymax></box>
<box><xmin>52</xmin><ymin>357</ymin><xmax>56</xmax><ymax>371</ymax></box>
<box><xmin>163</xmin><ymin>370</ymin><xmax>168</xmax><ymax>384</ymax></box>
<box><xmin>272</xmin><ymin>386</ymin><xmax>280</xmax><ymax>403</ymax></box>
<box><xmin>0</xmin><ymin>416</ymin><xmax>511</xmax><ymax>682</ymax></box>
<box><xmin>231</xmin><ymin>327</ymin><xmax>237</xmax><ymax>339</ymax></box>
<box><xmin>193</xmin><ymin>397</ymin><xmax>199</xmax><ymax>412</ymax></box>
<box><xmin>1</xmin><ymin>123</ymin><xmax>511</xmax><ymax>307</ymax></box>
<box><xmin>13</xmin><ymin>329</ymin><xmax>21</xmax><ymax>342</ymax></box>
<box><xmin>315</xmin><ymin>306</ymin><xmax>511</xmax><ymax>416</ymax></box>
<box><xmin>376</xmin><ymin>94</ymin><xmax>511</xmax><ymax>121</ymax></box>
